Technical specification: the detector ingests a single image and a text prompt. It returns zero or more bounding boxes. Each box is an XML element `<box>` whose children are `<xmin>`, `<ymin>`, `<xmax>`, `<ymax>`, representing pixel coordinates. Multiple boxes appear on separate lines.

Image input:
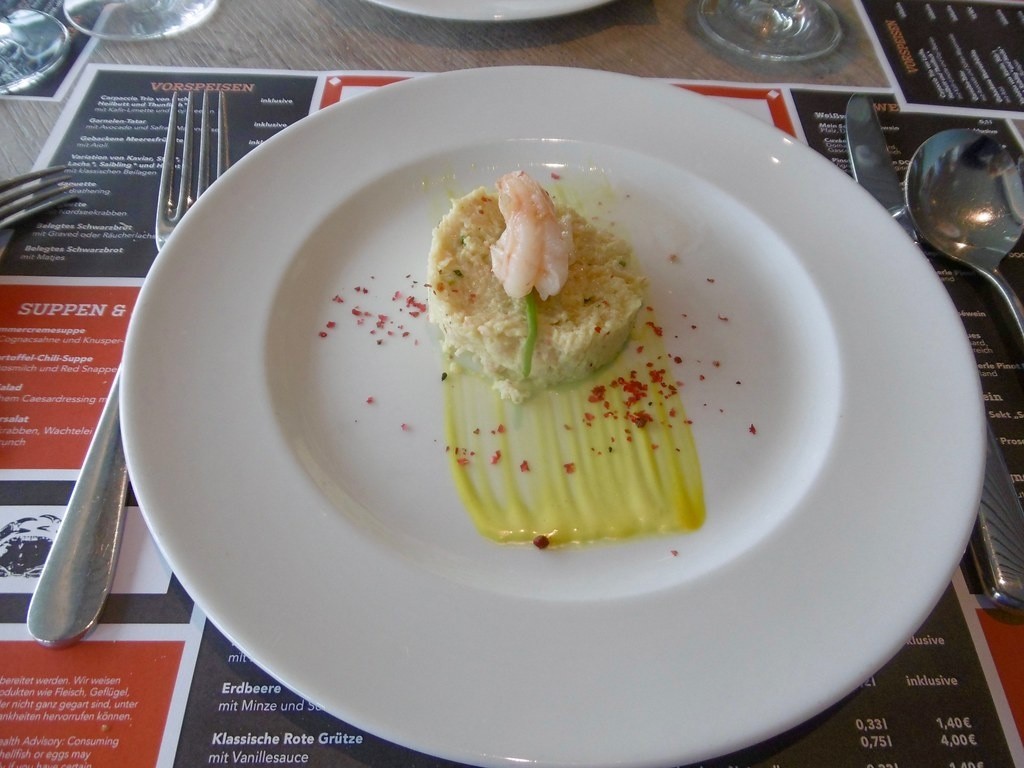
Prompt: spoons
<box><xmin>904</xmin><ymin>128</ymin><xmax>1023</xmax><ymax>346</ymax></box>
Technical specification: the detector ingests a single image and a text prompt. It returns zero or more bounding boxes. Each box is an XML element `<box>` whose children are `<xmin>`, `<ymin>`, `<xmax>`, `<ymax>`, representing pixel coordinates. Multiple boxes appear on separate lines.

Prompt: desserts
<box><xmin>429</xmin><ymin>170</ymin><xmax>643</xmax><ymax>390</ymax></box>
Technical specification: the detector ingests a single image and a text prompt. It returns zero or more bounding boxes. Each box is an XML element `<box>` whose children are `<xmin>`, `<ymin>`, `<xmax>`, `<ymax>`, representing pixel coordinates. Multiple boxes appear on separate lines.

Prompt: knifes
<box><xmin>843</xmin><ymin>92</ymin><xmax>1024</xmax><ymax>612</ymax></box>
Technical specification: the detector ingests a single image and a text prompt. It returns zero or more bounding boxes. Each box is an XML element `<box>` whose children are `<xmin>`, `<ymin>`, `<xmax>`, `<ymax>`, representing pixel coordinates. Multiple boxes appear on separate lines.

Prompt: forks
<box><xmin>0</xmin><ymin>165</ymin><xmax>79</xmax><ymax>229</ymax></box>
<box><xmin>22</xmin><ymin>89</ymin><xmax>229</xmax><ymax>645</ymax></box>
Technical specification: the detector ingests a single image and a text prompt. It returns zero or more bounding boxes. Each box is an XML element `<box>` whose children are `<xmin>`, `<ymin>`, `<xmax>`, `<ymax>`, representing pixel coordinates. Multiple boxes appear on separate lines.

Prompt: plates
<box><xmin>117</xmin><ymin>64</ymin><xmax>989</xmax><ymax>767</ymax></box>
<box><xmin>374</xmin><ymin>1</ymin><xmax>617</xmax><ymax>22</ymax></box>
